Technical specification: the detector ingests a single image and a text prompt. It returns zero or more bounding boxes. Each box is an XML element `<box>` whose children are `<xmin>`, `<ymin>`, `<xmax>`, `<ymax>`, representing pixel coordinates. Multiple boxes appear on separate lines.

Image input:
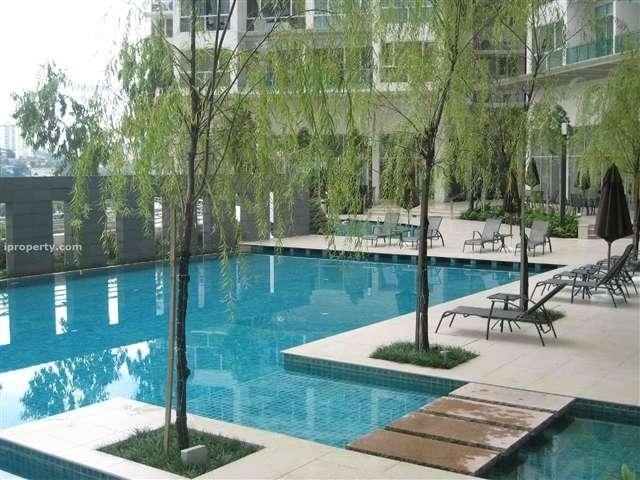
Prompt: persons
<box><xmin>476</xmin><ymin>177</ymin><xmax>483</xmax><ymax>205</ymax></box>
<box><xmin>206</xmin><ymin>0</ymin><xmax>215</xmax><ymax>30</ymax></box>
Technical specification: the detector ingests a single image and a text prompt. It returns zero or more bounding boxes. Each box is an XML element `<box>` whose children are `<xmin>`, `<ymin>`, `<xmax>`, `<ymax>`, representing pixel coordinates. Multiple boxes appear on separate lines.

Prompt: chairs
<box><xmin>462</xmin><ymin>218</ymin><xmax>514</xmax><ymax>256</ymax></box>
<box><xmin>514</xmin><ymin>220</ymin><xmax>552</xmax><ymax>258</ymax></box>
<box><xmin>363</xmin><ymin>213</ymin><xmax>445</xmax><ymax>251</ymax></box>
<box><xmin>435</xmin><ymin>282</ymin><xmax>568</xmax><ymax>346</ymax></box>
<box><xmin>531</xmin><ymin>244</ymin><xmax>639</xmax><ymax>308</ymax></box>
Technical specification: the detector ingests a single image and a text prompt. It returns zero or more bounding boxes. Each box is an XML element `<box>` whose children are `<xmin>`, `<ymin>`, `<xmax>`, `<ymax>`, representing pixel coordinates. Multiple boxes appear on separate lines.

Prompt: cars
<box><xmin>0</xmin><ymin>147</ymin><xmax>53</xmax><ymax>177</ymax></box>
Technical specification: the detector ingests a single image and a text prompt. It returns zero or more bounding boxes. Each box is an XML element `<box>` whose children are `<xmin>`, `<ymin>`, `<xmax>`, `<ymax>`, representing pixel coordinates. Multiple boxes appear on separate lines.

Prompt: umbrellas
<box><xmin>595</xmin><ymin>163</ymin><xmax>634</xmax><ymax>272</ymax></box>
<box><xmin>525</xmin><ymin>158</ymin><xmax>540</xmax><ymax>190</ymax></box>
<box><xmin>400</xmin><ymin>175</ymin><xmax>420</xmax><ymax>224</ymax></box>
<box><xmin>574</xmin><ymin>168</ymin><xmax>591</xmax><ymax>190</ymax></box>
<box><xmin>503</xmin><ymin>190</ymin><xmax>519</xmax><ymax>235</ymax></box>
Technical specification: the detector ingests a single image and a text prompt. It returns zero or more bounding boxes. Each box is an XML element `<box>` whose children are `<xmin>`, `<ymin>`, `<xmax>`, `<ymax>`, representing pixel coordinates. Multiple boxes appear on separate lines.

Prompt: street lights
<box><xmin>557</xmin><ymin>109</ymin><xmax>572</xmax><ymax>229</ymax></box>
